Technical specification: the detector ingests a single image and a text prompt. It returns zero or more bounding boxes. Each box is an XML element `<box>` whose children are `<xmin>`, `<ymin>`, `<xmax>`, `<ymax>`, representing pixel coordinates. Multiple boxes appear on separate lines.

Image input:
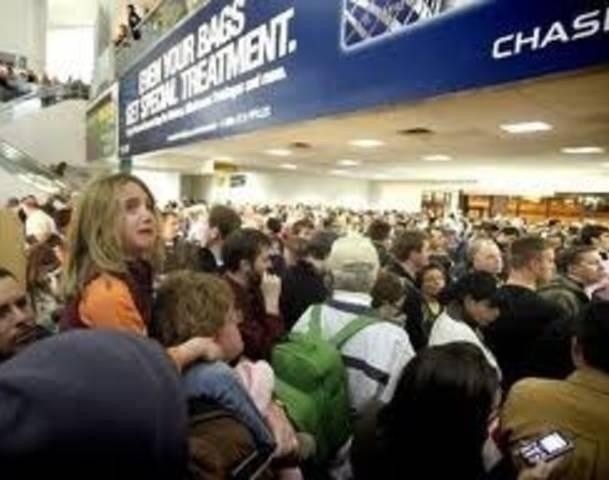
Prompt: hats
<box><xmin>330</xmin><ymin>236</ymin><xmax>380</xmax><ymax>273</ymax></box>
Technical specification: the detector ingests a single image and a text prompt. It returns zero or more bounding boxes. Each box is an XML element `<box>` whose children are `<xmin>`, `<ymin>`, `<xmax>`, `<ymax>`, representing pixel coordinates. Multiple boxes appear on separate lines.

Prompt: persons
<box><xmin>1</xmin><ymin>173</ymin><xmax>609</xmax><ymax>479</ymax></box>
<box><xmin>127</xmin><ymin>5</ymin><xmax>142</xmax><ymax>39</ymax></box>
<box><xmin>53</xmin><ymin>160</ymin><xmax>67</xmax><ymax>174</ymax></box>
<box><xmin>0</xmin><ymin>60</ymin><xmax>88</xmax><ymax>105</ymax></box>
<box><xmin>118</xmin><ymin>24</ymin><xmax>130</xmax><ymax>41</ymax></box>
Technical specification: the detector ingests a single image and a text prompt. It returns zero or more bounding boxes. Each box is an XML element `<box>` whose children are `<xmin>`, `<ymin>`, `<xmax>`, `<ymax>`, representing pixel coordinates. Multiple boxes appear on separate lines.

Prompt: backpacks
<box><xmin>271</xmin><ymin>306</ymin><xmax>382</xmax><ymax>462</ymax></box>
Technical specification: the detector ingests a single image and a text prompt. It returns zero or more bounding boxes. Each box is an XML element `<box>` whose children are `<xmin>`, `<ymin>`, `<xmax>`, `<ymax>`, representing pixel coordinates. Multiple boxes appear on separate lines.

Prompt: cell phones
<box><xmin>519</xmin><ymin>429</ymin><xmax>575</xmax><ymax>466</ymax></box>
